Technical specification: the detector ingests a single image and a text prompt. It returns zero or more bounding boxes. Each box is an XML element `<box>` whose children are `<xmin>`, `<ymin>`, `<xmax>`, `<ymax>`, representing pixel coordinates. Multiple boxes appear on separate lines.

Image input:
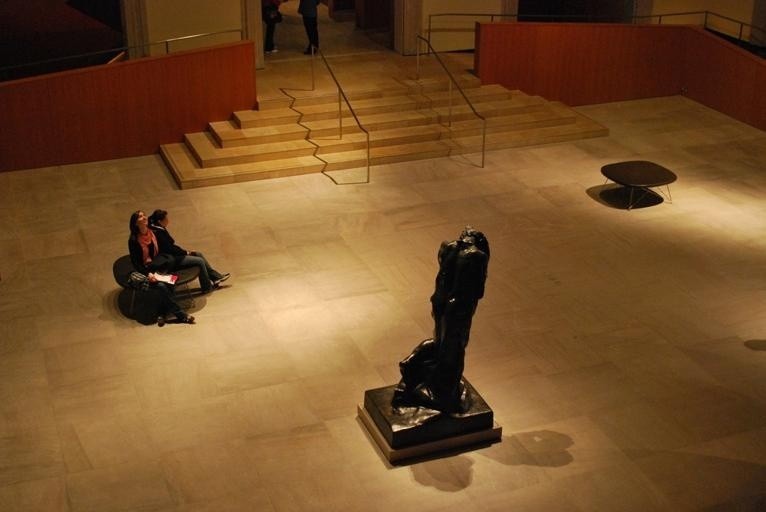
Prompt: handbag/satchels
<box><xmin>127</xmin><ymin>272</ymin><xmax>149</xmax><ymax>292</ymax></box>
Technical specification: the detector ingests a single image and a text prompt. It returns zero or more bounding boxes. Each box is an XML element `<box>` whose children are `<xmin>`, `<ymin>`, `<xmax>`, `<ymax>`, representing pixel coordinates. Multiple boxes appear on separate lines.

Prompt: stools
<box><xmin>112</xmin><ymin>255</ymin><xmax>200</xmax><ymax>314</ymax></box>
<box><xmin>601</xmin><ymin>161</ymin><xmax>676</xmax><ymax>209</ymax></box>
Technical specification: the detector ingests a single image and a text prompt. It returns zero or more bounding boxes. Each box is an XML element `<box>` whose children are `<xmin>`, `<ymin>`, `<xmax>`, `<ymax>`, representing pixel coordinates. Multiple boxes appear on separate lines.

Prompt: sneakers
<box><xmin>204</xmin><ymin>273</ymin><xmax>230</xmax><ymax>294</ymax></box>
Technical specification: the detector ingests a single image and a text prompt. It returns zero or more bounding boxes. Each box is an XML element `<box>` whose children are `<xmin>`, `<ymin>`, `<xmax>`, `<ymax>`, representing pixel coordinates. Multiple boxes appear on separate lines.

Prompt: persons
<box><xmin>147</xmin><ymin>209</ymin><xmax>230</xmax><ymax>294</ymax></box>
<box><xmin>262</xmin><ymin>0</ymin><xmax>281</xmax><ymax>54</ymax></box>
<box><xmin>399</xmin><ymin>229</ymin><xmax>489</xmax><ymax>394</ymax></box>
<box><xmin>298</xmin><ymin>0</ymin><xmax>320</xmax><ymax>55</ymax></box>
<box><xmin>128</xmin><ymin>209</ymin><xmax>194</xmax><ymax>326</ymax></box>
